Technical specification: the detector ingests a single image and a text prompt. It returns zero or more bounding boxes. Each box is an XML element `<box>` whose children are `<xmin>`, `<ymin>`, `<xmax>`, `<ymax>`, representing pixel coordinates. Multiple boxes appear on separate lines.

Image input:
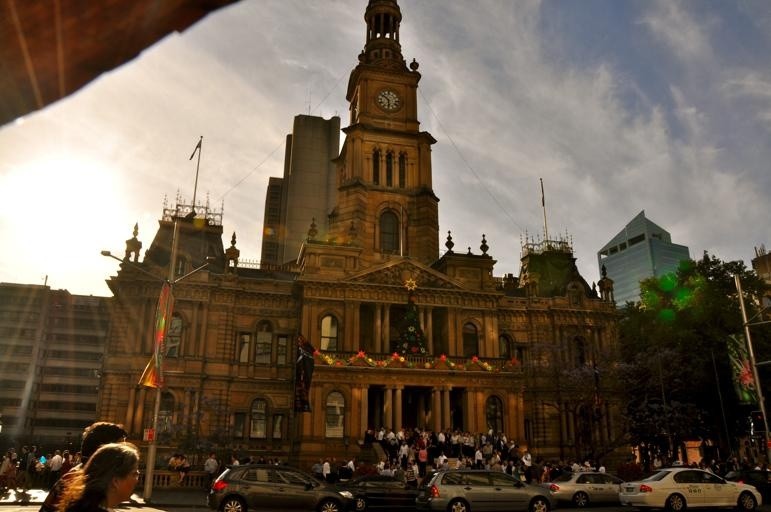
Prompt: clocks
<box><xmin>375</xmin><ymin>86</ymin><xmax>404</xmax><ymax>114</ymax></box>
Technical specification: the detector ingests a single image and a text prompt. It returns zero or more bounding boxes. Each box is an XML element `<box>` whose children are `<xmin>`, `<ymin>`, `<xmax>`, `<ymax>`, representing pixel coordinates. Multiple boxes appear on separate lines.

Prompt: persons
<box><xmin>1</xmin><ymin>418</ymin><xmax>771</xmax><ymax>512</ymax></box>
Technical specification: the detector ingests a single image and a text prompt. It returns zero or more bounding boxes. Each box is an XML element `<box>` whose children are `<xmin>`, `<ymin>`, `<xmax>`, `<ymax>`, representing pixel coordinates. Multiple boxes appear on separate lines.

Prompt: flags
<box><xmin>188</xmin><ymin>138</ymin><xmax>202</xmax><ymax>163</ymax></box>
<box><xmin>724</xmin><ymin>332</ymin><xmax>760</xmax><ymax>406</ymax></box>
<box><xmin>539</xmin><ymin>181</ymin><xmax>545</xmax><ymax>208</ymax></box>
<box><xmin>137</xmin><ymin>283</ymin><xmax>175</xmax><ymax>388</ymax></box>
<box><xmin>293</xmin><ymin>330</ymin><xmax>315</xmax><ymax>414</ymax></box>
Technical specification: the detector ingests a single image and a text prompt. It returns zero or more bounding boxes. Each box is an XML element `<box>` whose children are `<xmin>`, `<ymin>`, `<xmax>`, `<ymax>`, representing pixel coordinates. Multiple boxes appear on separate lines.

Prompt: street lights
<box><xmin>727</xmin><ymin>275</ymin><xmax>771</xmax><ymax>444</ymax></box>
<box><xmin>102</xmin><ymin>215</ymin><xmax>219</xmax><ymax>504</ymax></box>
